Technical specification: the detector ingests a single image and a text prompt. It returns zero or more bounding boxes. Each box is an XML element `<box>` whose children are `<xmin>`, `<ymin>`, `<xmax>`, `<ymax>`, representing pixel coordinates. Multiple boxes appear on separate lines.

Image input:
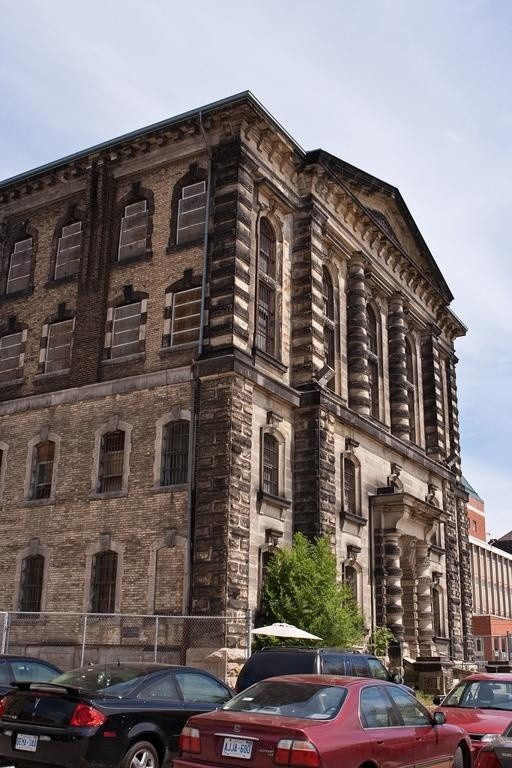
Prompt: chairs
<box><xmin>467</xmin><ymin>685</ymin><xmax>512</xmax><ymax>709</ymax></box>
<box><xmin>292</xmin><ymin>692</ymin><xmax>386</xmax><ymax>727</ymax></box>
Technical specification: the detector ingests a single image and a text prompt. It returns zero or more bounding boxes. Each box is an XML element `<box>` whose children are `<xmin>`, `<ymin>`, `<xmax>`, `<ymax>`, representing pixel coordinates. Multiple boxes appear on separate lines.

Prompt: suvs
<box><xmin>235</xmin><ymin>644</ymin><xmax>417</xmax><ymax>700</ymax></box>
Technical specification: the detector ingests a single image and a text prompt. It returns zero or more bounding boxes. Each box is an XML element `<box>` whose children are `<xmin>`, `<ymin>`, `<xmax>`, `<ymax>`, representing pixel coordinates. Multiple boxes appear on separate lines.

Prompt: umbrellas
<box><xmin>243</xmin><ymin>622</ymin><xmax>324</xmax><ymax>647</ymax></box>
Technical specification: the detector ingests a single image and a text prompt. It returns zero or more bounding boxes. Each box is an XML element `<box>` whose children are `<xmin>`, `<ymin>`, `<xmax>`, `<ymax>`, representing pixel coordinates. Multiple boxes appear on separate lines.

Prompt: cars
<box><xmin>1</xmin><ymin>661</ymin><xmax>235</xmax><ymax>768</ymax></box>
<box><xmin>170</xmin><ymin>673</ymin><xmax>476</xmax><ymax>768</ymax></box>
<box><xmin>0</xmin><ymin>653</ymin><xmax>84</xmax><ymax>700</ymax></box>
<box><xmin>429</xmin><ymin>672</ymin><xmax>512</xmax><ymax>768</ymax></box>
